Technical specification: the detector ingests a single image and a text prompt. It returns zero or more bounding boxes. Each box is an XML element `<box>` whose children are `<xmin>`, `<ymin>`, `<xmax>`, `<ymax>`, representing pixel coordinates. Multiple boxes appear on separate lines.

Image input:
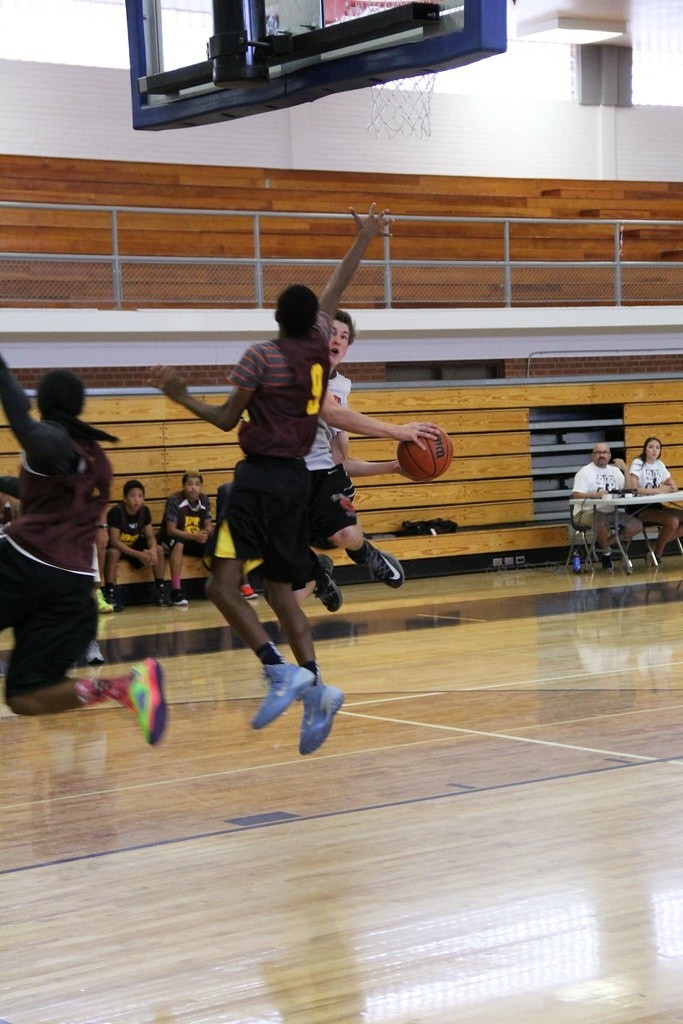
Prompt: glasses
<box><xmin>593</xmin><ymin>450</ymin><xmax>610</xmax><ymax>457</ymax></box>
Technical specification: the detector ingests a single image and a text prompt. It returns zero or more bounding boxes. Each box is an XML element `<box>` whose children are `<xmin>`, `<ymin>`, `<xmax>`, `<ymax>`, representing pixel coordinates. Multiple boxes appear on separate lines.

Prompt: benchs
<box><xmin>106</xmin><ymin>522</ymin><xmax>567</xmax><ymax>610</ymax></box>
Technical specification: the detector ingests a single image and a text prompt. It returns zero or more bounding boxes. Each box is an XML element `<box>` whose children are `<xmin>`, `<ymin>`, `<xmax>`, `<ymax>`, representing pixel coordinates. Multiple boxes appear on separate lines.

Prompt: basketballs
<box><xmin>397</xmin><ymin>423</ymin><xmax>455</xmax><ymax>484</ymax></box>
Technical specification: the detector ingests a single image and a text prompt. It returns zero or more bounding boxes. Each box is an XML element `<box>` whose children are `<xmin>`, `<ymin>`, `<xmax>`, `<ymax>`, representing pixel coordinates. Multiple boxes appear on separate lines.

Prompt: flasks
<box><xmin>573</xmin><ymin>554</ymin><xmax>581</xmax><ymax>573</ymax></box>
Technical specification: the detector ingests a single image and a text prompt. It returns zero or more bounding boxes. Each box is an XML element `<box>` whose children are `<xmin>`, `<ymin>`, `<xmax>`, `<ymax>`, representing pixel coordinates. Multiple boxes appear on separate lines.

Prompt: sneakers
<box><xmin>354</xmin><ymin>539</ymin><xmax>405</xmax><ymax>589</ymax></box>
<box><xmin>156</xmin><ymin>584</ymin><xmax>169</xmax><ymax>606</ymax></box>
<box><xmin>239</xmin><ymin>584</ymin><xmax>258</xmax><ymax>600</ymax></box>
<box><xmin>107</xmin><ymin>587</ymin><xmax>121</xmax><ymax>612</ymax></box>
<box><xmin>601</xmin><ymin>547</ymin><xmax>613</xmax><ymax>571</ymax></box>
<box><xmin>127</xmin><ymin>658</ymin><xmax>168</xmax><ymax>745</ymax></box>
<box><xmin>315</xmin><ymin>555</ymin><xmax>343</xmax><ymax>611</ymax></box>
<box><xmin>298</xmin><ymin>686</ymin><xmax>345</xmax><ymax>755</ymax></box>
<box><xmin>252</xmin><ymin>662</ymin><xmax>315</xmax><ymax>729</ymax></box>
<box><xmin>95</xmin><ymin>588</ymin><xmax>114</xmax><ymax>612</ymax></box>
<box><xmin>167</xmin><ymin>588</ymin><xmax>189</xmax><ymax>606</ymax></box>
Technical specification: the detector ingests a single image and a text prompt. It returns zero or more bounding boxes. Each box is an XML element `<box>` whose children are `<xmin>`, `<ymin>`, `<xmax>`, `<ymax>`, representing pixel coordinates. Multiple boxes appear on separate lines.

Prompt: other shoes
<box><xmin>644</xmin><ymin>540</ymin><xmax>663</xmax><ymax>567</ymax></box>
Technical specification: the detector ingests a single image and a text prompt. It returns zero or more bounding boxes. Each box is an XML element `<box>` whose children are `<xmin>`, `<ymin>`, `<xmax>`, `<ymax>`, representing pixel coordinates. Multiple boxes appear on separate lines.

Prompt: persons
<box><xmin>103</xmin><ymin>480</ymin><xmax>173</xmax><ymax>611</ymax></box>
<box><xmin>92</xmin><ymin>509</ymin><xmax>113</xmax><ymax>613</ymax></box>
<box><xmin>570</xmin><ymin>442</ymin><xmax>643</xmax><ymax>572</ymax></box>
<box><xmin>628</xmin><ymin>437</ymin><xmax>683</xmax><ymax>569</ymax></box>
<box><xmin>159</xmin><ymin>465</ymin><xmax>214</xmax><ymax>606</ymax></box>
<box><xmin>217</xmin><ymin>459</ymin><xmax>258</xmax><ymax>600</ymax></box>
<box><xmin>147</xmin><ymin>203</ymin><xmax>395</xmax><ymax>755</ymax></box>
<box><xmin>0</xmin><ymin>351</ymin><xmax>168</xmax><ymax>745</ymax></box>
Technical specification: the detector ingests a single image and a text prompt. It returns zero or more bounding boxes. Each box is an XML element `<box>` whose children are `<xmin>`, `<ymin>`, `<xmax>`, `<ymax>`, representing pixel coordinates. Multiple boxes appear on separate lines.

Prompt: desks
<box><xmin>569</xmin><ymin>490</ymin><xmax>683</xmax><ymax>575</ymax></box>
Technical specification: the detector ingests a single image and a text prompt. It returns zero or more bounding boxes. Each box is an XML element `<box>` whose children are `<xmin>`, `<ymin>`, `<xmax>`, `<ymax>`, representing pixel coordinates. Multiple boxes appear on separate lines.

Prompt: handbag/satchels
<box><xmin>401</xmin><ymin>518</ymin><xmax>458</xmax><ymax>535</ymax></box>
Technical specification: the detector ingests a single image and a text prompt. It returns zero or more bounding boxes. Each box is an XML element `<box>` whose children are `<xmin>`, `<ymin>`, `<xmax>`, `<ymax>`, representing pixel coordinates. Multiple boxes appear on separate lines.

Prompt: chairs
<box><xmin>623</xmin><ymin>508</ymin><xmax>681</xmax><ymax>570</ymax></box>
<box><xmin>570</xmin><ymin>503</ymin><xmax>629</xmax><ymax>572</ymax></box>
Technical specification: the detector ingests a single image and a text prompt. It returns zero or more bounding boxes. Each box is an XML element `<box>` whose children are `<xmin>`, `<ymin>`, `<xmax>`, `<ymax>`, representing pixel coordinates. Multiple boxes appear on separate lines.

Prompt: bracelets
<box><xmin>97</xmin><ymin>524</ymin><xmax>109</xmax><ymax>529</ymax></box>
